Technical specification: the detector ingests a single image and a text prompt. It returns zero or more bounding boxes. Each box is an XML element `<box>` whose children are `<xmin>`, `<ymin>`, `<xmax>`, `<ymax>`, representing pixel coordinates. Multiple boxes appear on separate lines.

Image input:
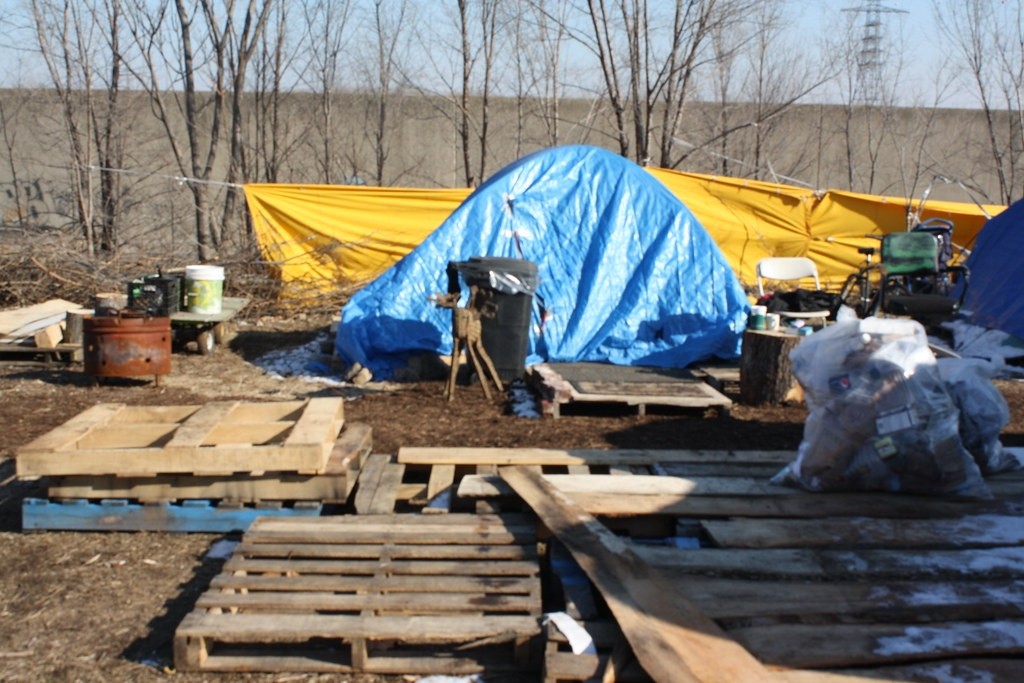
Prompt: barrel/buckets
<box><xmin>186</xmin><ymin>265</ymin><xmax>224</xmax><ymax>315</ymax></box>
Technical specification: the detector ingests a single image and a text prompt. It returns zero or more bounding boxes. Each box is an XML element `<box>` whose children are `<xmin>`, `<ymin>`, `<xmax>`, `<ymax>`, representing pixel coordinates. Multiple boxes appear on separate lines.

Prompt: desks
<box><xmin>169</xmin><ymin>298</ymin><xmax>252</xmax><ymax>353</ymax></box>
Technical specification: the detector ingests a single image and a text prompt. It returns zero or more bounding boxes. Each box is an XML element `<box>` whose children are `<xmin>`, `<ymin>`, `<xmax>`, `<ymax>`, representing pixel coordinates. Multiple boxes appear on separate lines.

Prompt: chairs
<box><xmin>756</xmin><ymin>257</ymin><xmax>831</xmax><ymax>328</ymax></box>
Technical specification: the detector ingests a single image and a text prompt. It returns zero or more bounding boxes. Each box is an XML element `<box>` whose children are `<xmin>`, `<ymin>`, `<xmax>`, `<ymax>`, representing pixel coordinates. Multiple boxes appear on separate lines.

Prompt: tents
<box><xmin>941</xmin><ymin>197</ymin><xmax>1024</xmax><ymax>367</ymax></box>
<box><xmin>333</xmin><ymin>146</ymin><xmax>755</xmax><ymax>380</ymax></box>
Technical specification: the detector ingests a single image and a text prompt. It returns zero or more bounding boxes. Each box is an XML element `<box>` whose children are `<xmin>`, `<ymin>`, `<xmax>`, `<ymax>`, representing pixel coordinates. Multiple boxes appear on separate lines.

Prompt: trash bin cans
<box><xmin>448</xmin><ymin>256</ymin><xmax>538</xmax><ymax>387</ymax></box>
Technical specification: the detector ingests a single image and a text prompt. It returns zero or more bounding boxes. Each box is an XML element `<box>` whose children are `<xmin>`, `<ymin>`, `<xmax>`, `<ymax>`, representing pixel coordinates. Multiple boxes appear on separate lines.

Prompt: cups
<box><xmin>751</xmin><ymin>305</ymin><xmax>767</xmax><ymax>330</ymax></box>
<box><xmin>766</xmin><ymin>313</ymin><xmax>780</xmax><ymax>329</ymax></box>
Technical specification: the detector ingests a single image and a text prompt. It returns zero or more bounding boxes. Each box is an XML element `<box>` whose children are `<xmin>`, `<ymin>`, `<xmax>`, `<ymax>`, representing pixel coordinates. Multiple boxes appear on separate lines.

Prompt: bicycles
<box><xmin>840</xmin><ymin>235</ymin><xmax>911</xmax><ymax>319</ymax></box>
